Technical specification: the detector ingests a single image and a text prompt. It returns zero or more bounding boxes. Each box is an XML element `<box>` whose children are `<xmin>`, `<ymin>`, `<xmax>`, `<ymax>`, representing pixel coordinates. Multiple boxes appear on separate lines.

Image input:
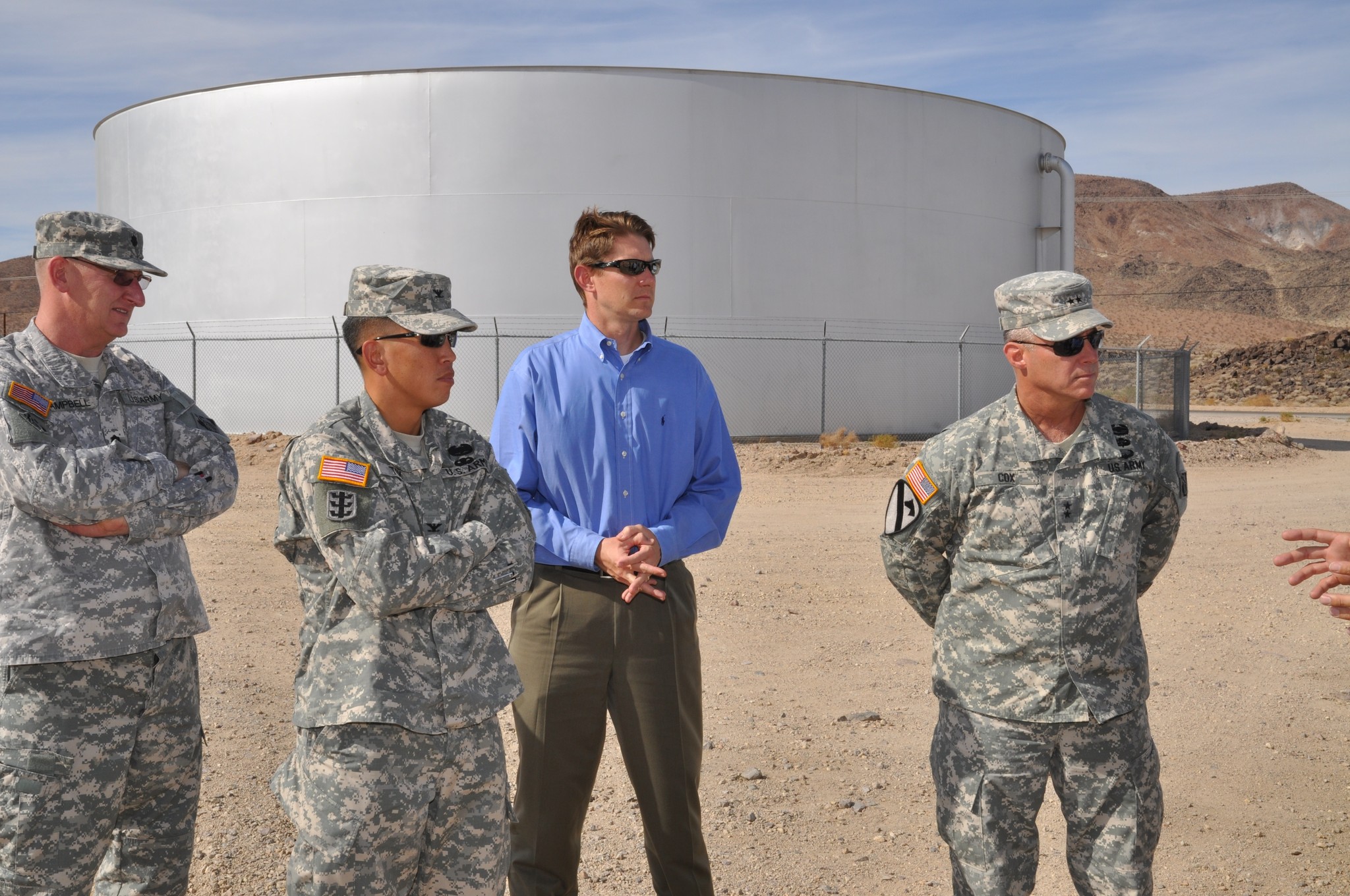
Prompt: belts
<box><xmin>561</xmin><ymin>564</ymin><xmax>613</xmax><ymax>578</ymax></box>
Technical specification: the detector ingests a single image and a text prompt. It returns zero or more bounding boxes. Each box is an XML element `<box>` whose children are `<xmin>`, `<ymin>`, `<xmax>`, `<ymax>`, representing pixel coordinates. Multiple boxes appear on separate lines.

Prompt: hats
<box><xmin>994</xmin><ymin>271</ymin><xmax>1114</xmax><ymax>342</ymax></box>
<box><xmin>32</xmin><ymin>212</ymin><xmax>168</xmax><ymax>277</ymax></box>
<box><xmin>342</xmin><ymin>266</ymin><xmax>478</xmax><ymax>335</ymax></box>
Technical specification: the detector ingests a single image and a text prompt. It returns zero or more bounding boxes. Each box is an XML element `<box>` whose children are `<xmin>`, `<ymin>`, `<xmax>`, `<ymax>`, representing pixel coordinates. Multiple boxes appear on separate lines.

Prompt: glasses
<box><xmin>64</xmin><ymin>256</ymin><xmax>152</xmax><ymax>289</ymax></box>
<box><xmin>586</xmin><ymin>259</ymin><xmax>662</xmax><ymax>276</ymax></box>
<box><xmin>1013</xmin><ymin>329</ymin><xmax>1105</xmax><ymax>357</ymax></box>
<box><xmin>355</xmin><ymin>329</ymin><xmax>458</xmax><ymax>355</ymax></box>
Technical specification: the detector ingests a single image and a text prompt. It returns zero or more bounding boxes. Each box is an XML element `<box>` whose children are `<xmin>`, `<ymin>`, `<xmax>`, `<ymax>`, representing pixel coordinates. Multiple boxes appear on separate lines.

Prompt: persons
<box><xmin>488</xmin><ymin>211</ymin><xmax>741</xmax><ymax>896</ymax></box>
<box><xmin>1273</xmin><ymin>528</ymin><xmax>1350</xmax><ymax>635</ymax></box>
<box><xmin>0</xmin><ymin>210</ymin><xmax>239</xmax><ymax>896</ymax></box>
<box><xmin>879</xmin><ymin>270</ymin><xmax>1188</xmax><ymax>896</ymax></box>
<box><xmin>269</xmin><ymin>264</ymin><xmax>538</xmax><ymax>896</ymax></box>
